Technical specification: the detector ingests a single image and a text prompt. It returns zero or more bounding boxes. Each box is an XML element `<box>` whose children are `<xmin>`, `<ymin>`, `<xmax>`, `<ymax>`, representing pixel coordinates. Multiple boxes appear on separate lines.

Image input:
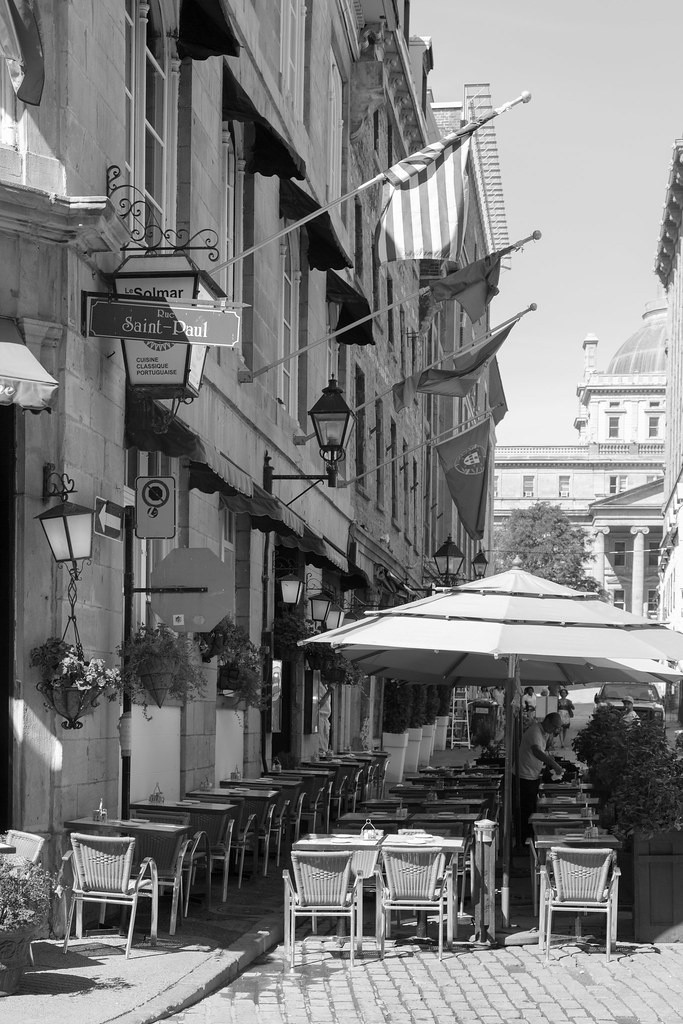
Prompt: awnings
<box><xmin>0</xmin><ymin>317</ymin><xmax>59</xmax><ymax>415</ymax></box>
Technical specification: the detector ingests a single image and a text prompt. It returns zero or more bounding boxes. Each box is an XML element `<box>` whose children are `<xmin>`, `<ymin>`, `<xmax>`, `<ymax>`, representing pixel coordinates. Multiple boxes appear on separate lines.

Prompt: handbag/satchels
<box><xmin>568</xmin><ymin>700</ymin><xmax>573</xmax><ymax>717</ymax></box>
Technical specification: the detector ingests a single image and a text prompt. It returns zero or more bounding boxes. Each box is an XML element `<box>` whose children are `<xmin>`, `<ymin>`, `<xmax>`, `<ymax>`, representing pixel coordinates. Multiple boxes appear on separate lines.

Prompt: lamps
<box><xmin>402</xmin><ymin>530</ymin><xmax>466</xmax><ymax>594</ymax></box>
<box><xmin>260</xmin><ymin>372</ymin><xmax>355</xmax><ymax>498</ymax></box>
<box><xmin>258</xmin><ymin>550</ymin><xmax>355</xmax><ymax>632</ymax></box>
<box><xmin>34</xmin><ymin>464</ymin><xmax>94</xmax><ymax>582</ymax></box>
<box><xmin>99</xmin><ymin>163</ymin><xmax>229</xmax><ymax>435</ymax></box>
<box><xmin>455</xmin><ymin>549</ymin><xmax>490</xmax><ymax>582</ymax></box>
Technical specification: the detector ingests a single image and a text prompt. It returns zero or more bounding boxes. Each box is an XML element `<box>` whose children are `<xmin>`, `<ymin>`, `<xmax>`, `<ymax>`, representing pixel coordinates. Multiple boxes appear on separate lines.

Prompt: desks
<box><xmin>65</xmin><ymin>742</ymin><xmax>508</xmax><ymax>938</ymax></box>
<box><xmin>524</xmin><ymin>782</ymin><xmax>623</xmax><ymax>917</ymax></box>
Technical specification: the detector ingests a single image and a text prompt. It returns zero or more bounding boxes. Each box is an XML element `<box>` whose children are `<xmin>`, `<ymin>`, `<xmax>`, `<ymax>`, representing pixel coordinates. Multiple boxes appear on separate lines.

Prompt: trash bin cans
<box><xmin>470</xmin><ymin>700</ymin><xmax>499</xmax><ymax>746</ymax></box>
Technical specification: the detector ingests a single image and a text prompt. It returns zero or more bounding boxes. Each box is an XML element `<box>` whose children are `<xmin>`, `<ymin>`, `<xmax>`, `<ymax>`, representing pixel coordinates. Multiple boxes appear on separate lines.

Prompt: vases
<box><xmin>0</xmin><ymin>925</ymin><xmax>39</xmax><ymax>997</ymax></box>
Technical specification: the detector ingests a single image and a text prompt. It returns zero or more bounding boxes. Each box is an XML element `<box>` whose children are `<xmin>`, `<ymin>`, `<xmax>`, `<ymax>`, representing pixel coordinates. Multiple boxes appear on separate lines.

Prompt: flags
<box><xmin>434</xmin><ymin>418</ymin><xmax>493</xmax><ymax>541</ymax></box>
<box><xmin>427</xmin><ymin>242</ymin><xmax>499</xmax><ymax>322</ymax></box>
<box><xmin>379</xmin><ymin>102</ymin><xmax>511</xmax><ymax>262</ymax></box>
<box><xmin>391</xmin><ymin>318</ymin><xmax>520</xmax><ymax>415</ymax></box>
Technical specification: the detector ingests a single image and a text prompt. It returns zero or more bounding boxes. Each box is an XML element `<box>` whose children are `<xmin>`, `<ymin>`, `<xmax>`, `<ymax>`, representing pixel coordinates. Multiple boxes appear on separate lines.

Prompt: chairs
<box><xmin>4</xmin><ymin>829</ymin><xmax>46</xmax><ymax>866</ymax></box>
<box><xmin>535</xmin><ymin>846</ymin><xmax>622</xmax><ymax>962</ymax></box>
<box><xmin>62</xmin><ymin>755</ymin><xmax>452</xmax><ymax>967</ymax></box>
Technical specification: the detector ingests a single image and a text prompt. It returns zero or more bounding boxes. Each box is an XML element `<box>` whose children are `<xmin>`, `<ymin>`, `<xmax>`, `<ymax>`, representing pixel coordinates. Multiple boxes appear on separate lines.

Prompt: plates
<box><xmin>564</xmin><ymin>834</ymin><xmax>585</xmax><ymax>841</ymax></box>
<box><xmin>122</xmin><ymin>819</ymin><xmax>149</xmax><ymax>827</ymax></box>
<box><xmin>409</xmin><ymin>835</ymin><xmax>432</xmax><ymax>845</ymax></box>
<box><xmin>257</xmin><ymin>778</ymin><xmax>273</xmax><ymax>782</ymax></box>
<box><xmin>369</xmin><ymin>812</ymin><xmax>387</xmax><ymax>817</ymax></box>
<box><xmin>445</xmin><ymin>798</ymin><xmax>462</xmax><ymax>803</ymax></box>
<box><xmin>557</xmin><ymin>796</ymin><xmax>572</xmax><ymax>803</ymax></box>
<box><xmin>229</xmin><ymin>787</ymin><xmax>250</xmax><ymax>793</ymax></box>
<box><xmin>438</xmin><ymin>812</ymin><xmax>455</xmax><ymax>819</ymax></box>
<box><xmin>552</xmin><ymin>812</ymin><xmax>569</xmax><ymax>817</ymax></box>
<box><xmin>386</xmin><ymin>797</ymin><xmax>403</xmax><ymax>801</ymax></box>
<box><xmin>332</xmin><ymin>834</ymin><xmax>354</xmax><ymax>843</ymax></box>
<box><xmin>558</xmin><ymin>782</ymin><xmax>571</xmax><ymax>788</ymax></box>
<box><xmin>410</xmin><ymin>785</ymin><xmax>424</xmax><ymax>789</ymax></box>
<box><xmin>176</xmin><ymin>799</ymin><xmax>200</xmax><ymax>806</ymax></box>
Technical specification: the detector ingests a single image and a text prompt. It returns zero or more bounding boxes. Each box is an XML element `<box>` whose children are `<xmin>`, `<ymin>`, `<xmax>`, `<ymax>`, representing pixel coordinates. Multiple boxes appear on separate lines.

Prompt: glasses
<box><xmin>550</xmin><ymin>719</ymin><xmax>559</xmax><ymax>729</ymax></box>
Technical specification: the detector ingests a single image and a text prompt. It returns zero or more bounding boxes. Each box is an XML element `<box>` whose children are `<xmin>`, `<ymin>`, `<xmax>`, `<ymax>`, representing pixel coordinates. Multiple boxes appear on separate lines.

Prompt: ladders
<box><xmin>451</xmin><ymin>687</ymin><xmax>471</xmax><ymax>751</ymax></box>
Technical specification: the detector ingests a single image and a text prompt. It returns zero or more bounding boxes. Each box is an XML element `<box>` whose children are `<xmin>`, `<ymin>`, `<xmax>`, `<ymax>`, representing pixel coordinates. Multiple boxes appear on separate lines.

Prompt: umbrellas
<box><xmin>298</xmin><ymin>553</ymin><xmax>683</xmax><ymax>946</ymax></box>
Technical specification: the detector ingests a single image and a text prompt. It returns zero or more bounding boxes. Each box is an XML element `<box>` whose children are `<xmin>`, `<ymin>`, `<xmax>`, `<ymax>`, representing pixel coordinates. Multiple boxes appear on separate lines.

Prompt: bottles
<box><xmin>272</xmin><ymin>761</ymin><xmax>281</xmax><ymax>771</ymax></box>
<box><xmin>437</xmin><ymin>780</ymin><xmax>444</xmax><ymax>788</ymax></box>
<box><xmin>397</xmin><ymin>808</ymin><xmax>407</xmax><ymax>816</ymax></box>
<box><xmin>446</xmin><ymin>770</ymin><xmax>453</xmax><ymax>778</ymax></box>
<box><xmin>586</xmin><ymin>827</ymin><xmax>598</xmax><ymax>838</ymax></box>
<box><xmin>201</xmin><ymin>782</ymin><xmax>212</xmax><ymax>791</ymax></box>
<box><xmin>231</xmin><ymin>772</ymin><xmax>241</xmax><ymax>780</ymax></box>
<box><xmin>571</xmin><ymin>779</ymin><xmax>580</xmax><ymax>788</ymax></box>
<box><xmin>312</xmin><ymin>750</ymin><xmax>333</xmax><ymax>761</ymax></box>
<box><xmin>364</xmin><ymin>830</ymin><xmax>376</xmax><ymax>840</ymax></box>
<box><xmin>577</xmin><ymin>792</ymin><xmax>586</xmax><ymax>801</ymax></box>
<box><xmin>428</xmin><ymin>792</ymin><xmax>437</xmax><ymax>800</ymax></box>
<box><xmin>149</xmin><ymin>792</ymin><xmax>164</xmax><ymax>803</ymax></box>
<box><xmin>93</xmin><ymin>809</ymin><xmax>107</xmax><ymax>821</ymax></box>
<box><xmin>581</xmin><ymin>808</ymin><xmax>595</xmax><ymax>816</ymax></box>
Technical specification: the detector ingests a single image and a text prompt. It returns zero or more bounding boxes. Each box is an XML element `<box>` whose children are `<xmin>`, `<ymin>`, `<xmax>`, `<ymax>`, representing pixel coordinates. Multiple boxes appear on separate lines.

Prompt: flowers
<box><xmin>0</xmin><ymin>852</ymin><xmax>71</xmax><ymax>930</ymax></box>
<box><xmin>29</xmin><ymin>637</ymin><xmax>122</xmax><ymax>704</ymax></box>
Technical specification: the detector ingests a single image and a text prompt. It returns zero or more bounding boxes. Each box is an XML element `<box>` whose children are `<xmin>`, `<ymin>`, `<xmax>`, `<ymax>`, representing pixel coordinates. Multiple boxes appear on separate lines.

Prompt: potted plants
<box><xmin>219</xmin><ymin>632</ymin><xmax>281</xmax><ymax>726</ymax></box>
<box><xmin>114</xmin><ymin>622</ymin><xmax>207</xmax><ymax>721</ymax></box>
<box><xmin>382</xmin><ymin>678</ymin><xmax>451</xmax><ymax>782</ymax></box>
<box><xmin>272</xmin><ymin>604</ymin><xmax>361</xmax><ymax>694</ymax></box>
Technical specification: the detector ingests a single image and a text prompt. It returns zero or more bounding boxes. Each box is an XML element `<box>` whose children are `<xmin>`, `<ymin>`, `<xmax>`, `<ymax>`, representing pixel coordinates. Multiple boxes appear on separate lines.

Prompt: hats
<box><xmin>622</xmin><ymin>696</ymin><xmax>634</xmax><ymax>704</ymax></box>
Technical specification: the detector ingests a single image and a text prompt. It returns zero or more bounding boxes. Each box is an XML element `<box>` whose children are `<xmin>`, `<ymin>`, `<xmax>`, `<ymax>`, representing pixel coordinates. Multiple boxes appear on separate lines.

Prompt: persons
<box><xmin>491</xmin><ymin>686</ymin><xmax>504</xmax><ymax>731</ymax></box>
<box><xmin>512</xmin><ymin>711</ymin><xmax>562</xmax><ymax>851</ymax></box>
<box><xmin>522</xmin><ymin>686</ymin><xmax>536</xmax><ymax>711</ymax></box>
<box><xmin>320</xmin><ymin>674</ymin><xmax>335</xmax><ymax>752</ymax></box>
<box><xmin>558</xmin><ymin>689</ymin><xmax>575</xmax><ymax>748</ymax></box>
<box><xmin>547</xmin><ymin>686</ymin><xmax>561</xmax><ymax>706</ymax></box>
<box><xmin>477</xmin><ymin>686</ymin><xmax>491</xmax><ymax>699</ymax></box>
<box><xmin>619</xmin><ymin>696</ymin><xmax>640</xmax><ymax>730</ymax></box>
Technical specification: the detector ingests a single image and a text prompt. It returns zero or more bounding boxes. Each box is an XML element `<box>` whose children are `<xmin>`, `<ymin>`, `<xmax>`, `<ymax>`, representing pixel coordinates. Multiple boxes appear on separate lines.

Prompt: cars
<box><xmin>593</xmin><ymin>681</ymin><xmax>667</xmax><ymax>740</ymax></box>
